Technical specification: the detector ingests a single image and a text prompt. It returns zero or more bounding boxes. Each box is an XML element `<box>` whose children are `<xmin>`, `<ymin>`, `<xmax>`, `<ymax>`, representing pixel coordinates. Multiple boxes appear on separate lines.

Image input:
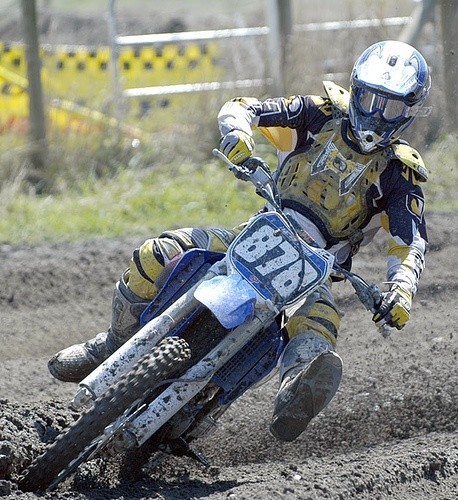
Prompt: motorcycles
<box><xmin>17</xmin><ymin>136</ymin><xmax>405</xmax><ymax>493</ymax></box>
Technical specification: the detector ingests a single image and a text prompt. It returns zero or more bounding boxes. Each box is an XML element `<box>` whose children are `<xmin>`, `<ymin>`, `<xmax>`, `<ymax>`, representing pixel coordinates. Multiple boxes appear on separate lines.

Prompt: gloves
<box><xmin>373</xmin><ymin>288</ymin><xmax>411</xmax><ymax>327</ymax></box>
<box><xmin>219</xmin><ymin>130</ymin><xmax>255</xmax><ymax>164</ymax></box>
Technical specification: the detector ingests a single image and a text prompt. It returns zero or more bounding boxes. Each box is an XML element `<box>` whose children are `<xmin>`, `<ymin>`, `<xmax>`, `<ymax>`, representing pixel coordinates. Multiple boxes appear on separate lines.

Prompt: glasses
<box><xmin>352</xmin><ymin>84</ymin><xmax>409</xmax><ymax>122</ymax></box>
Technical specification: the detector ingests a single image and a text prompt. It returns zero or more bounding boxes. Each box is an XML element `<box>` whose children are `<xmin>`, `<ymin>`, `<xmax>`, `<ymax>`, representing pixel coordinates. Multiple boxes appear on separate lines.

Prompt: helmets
<box><xmin>349</xmin><ymin>41</ymin><xmax>430</xmax><ymax>151</ymax></box>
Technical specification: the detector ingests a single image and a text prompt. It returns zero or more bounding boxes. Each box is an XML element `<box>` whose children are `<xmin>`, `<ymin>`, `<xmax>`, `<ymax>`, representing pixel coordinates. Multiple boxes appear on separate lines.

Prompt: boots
<box><xmin>270</xmin><ymin>333</ymin><xmax>343</xmax><ymax>442</ymax></box>
<box><xmin>48</xmin><ymin>268</ymin><xmax>149</xmax><ymax>382</ymax></box>
